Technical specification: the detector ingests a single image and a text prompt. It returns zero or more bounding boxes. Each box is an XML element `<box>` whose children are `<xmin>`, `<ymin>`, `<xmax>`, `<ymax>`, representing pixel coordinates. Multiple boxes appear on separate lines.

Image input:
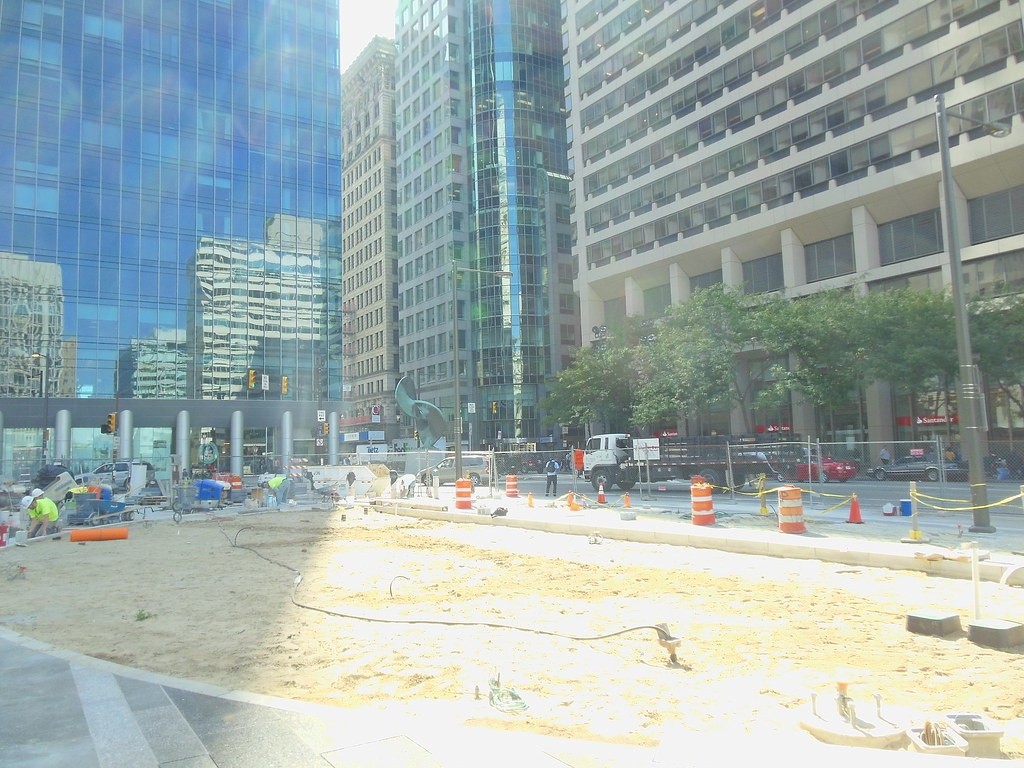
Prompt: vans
<box><xmin>416</xmin><ymin>456</ymin><xmax>492</xmax><ymax>487</ymax></box>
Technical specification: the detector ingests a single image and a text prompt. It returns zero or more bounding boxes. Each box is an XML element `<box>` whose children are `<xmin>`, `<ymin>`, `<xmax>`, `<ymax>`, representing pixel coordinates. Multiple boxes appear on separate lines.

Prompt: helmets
<box><xmin>32</xmin><ymin>488</ymin><xmax>44</xmax><ymax>498</ymax></box>
<box><xmin>21</xmin><ymin>496</ymin><xmax>34</xmax><ymax>508</ymax></box>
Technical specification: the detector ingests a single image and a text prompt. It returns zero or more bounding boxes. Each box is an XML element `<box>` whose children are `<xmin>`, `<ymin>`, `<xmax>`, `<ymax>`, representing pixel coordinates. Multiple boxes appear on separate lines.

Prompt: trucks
<box><xmin>582</xmin><ymin>434</ymin><xmax>805</xmax><ymax>492</ymax></box>
<box><xmin>12</xmin><ymin>445</ymin><xmax>49</xmax><ymax>483</ymax></box>
<box><xmin>347</xmin><ymin>444</ymin><xmax>393</xmax><ymax>466</ymax></box>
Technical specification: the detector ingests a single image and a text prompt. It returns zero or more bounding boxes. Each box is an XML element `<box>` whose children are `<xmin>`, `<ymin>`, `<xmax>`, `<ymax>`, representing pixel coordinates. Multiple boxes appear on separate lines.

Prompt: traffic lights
<box><xmin>397</xmin><ymin>416</ymin><xmax>401</xmax><ymax>422</ymax></box>
<box><xmin>281</xmin><ymin>377</ymin><xmax>288</xmax><ymax>394</ymax></box>
<box><xmin>414</xmin><ymin>430</ymin><xmax>418</xmax><ymax>440</ymax></box>
<box><xmin>324</xmin><ymin>422</ymin><xmax>329</xmax><ymax>436</ymax></box>
<box><xmin>248</xmin><ymin>369</ymin><xmax>256</xmax><ymax>390</ymax></box>
<box><xmin>493</xmin><ymin>402</ymin><xmax>499</xmax><ymax>414</ymax></box>
<box><xmin>107</xmin><ymin>414</ymin><xmax>115</xmax><ymax>432</ymax></box>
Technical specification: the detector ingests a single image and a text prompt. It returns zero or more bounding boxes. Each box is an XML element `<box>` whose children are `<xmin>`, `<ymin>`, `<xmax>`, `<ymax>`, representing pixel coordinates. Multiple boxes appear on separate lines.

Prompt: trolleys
<box><xmin>86</xmin><ymin>499</ymin><xmax>154</xmax><ymax>525</ymax></box>
<box><xmin>315</xmin><ymin>484</ymin><xmax>340</xmax><ymax>502</ymax></box>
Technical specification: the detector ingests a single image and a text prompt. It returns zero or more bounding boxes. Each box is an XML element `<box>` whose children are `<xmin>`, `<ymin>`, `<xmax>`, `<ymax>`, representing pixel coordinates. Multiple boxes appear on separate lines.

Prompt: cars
<box><xmin>773</xmin><ymin>448</ymin><xmax>859</xmax><ymax>482</ymax></box>
<box><xmin>74</xmin><ymin>462</ymin><xmax>156</xmax><ymax>491</ymax></box>
<box><xmin>865</xmin><ymin>456</ymin><xmax>966</xmax><ymax>482</ymax></box>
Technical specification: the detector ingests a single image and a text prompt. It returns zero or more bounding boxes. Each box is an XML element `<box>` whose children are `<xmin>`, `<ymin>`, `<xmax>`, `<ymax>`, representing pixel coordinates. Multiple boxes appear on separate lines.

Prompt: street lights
<box><xmin>452</xmin><ymin>259</ymin><xmax>514</xmax><ymax>481</ymax></box>
<box><xmin>31</xmin><ymin>353</ymin><xmax>52</xmax><ymax>466</ymax></box>
<box><xmin>935</xmin><ymin>94</ymin><xmax>1012</xmax><ymax>535</ymax></box>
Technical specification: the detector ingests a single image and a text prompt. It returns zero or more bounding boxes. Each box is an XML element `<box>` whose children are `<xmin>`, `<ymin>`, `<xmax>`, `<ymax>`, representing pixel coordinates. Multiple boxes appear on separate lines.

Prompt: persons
<box><xmin>546</xmin><ymin>455</ymin><xmax>559</xmax><ymax>497</ymax></box>
<box><xmin>262</xmin><ymin>475</ymin><xmax>290</xmax><ymax>507</ymax></box>
<box><xmin>945</xmin><ymin>447</ymin><xmax>956</xmax><ymax>463</ymax></box>
<box><xmin>879</xmin><ymin>444</ymin><xmax>890</xmax><ymax>466</ymax></box>
<box><xmin>21</xmin><ymin>488</ymin><xmax>59</xmax><ymax>540</ymax></box>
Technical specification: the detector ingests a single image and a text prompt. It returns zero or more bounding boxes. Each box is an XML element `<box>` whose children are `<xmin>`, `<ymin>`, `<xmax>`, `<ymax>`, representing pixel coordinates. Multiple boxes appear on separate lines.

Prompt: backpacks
<box><xmin>547</xmin><ymin>460</ymin><xmax>555</xmax><ymax>472</ymax></box>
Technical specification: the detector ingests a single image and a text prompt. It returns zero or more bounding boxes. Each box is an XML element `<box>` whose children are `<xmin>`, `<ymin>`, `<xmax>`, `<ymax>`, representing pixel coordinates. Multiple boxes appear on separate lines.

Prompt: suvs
<box><xmin>505</xmin><ymin>452</ymin><xmax>546</xmax><ymax>474</ymax></box>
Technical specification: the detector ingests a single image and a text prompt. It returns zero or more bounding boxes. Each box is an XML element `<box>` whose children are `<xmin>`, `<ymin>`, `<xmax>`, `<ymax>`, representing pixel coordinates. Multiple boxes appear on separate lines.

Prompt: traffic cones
<box><xmin>845</xmin><ymin>493</ymin><xmax>865</xmax><ymax>524</ymax></box>
<box><xmin>526</xmin><ymin>482</ymin><xmax>631</xmax><ymax>512</ymax></box>
